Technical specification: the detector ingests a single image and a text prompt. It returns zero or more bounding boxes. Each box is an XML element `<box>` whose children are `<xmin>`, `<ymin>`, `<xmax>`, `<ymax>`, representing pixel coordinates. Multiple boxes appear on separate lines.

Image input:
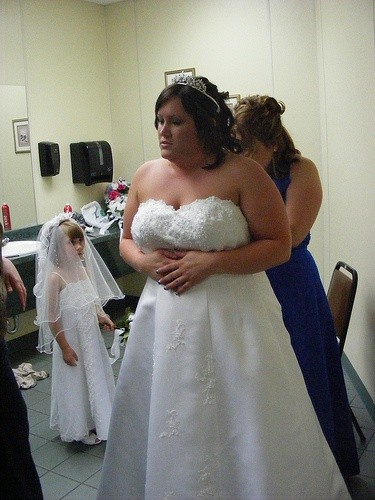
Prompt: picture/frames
<box><xmin>12</xmin><ymin>118</ymin><xmax>31</xmax><ymax>154</ymax></box>
<box><xmin>164</xmin><ymin>68</ymin><xmax>195</xmax><ymax>88</ymax></box>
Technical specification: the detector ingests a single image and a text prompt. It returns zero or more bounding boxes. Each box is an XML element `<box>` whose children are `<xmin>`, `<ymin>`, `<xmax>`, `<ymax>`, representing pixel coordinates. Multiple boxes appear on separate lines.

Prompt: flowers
<box><xmin>112</xmin><ymin>307</ymin><xmax>136</xmax><ymax>344</ymax></box>
<box><xmin>105</xmin><ymin>178</ymin><xmax>130</xmax><ymax>219</ymax></box>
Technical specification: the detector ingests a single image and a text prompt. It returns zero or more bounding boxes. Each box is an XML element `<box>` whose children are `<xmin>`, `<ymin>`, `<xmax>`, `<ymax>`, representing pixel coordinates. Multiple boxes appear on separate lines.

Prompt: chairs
<box><xmin>321</xmin><ymin>260</ymin><xmax>366</xmax><ymax>444</ymax></box>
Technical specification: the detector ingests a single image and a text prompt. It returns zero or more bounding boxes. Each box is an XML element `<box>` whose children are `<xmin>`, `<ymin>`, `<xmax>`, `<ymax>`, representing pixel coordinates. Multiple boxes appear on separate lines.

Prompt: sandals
<box><xmin>81</xmin><ymin>433</ymin><xmax>102</xmax><ymax>444</ymax></box>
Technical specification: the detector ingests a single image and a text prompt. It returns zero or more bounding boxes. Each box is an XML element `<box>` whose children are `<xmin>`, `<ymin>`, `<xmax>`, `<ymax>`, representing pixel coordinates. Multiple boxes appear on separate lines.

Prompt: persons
<box><xmin>33</xmin><ymin>213</ymin><xmax>126</xmax><ymax>445</ymax></box>
<box><xmin>97</xmin><ymin>70</ymin><xmax>353</xmax><ymax>500</ymax></box>
<box><xmin>230</xmin><ymin>95</ymin><xmax>361</xmax><ymax>475</ymax></box>
<box><xmin>0</xmin><ymin>223</ymin><xmax>44</xmax><ymax>500</ymax></box>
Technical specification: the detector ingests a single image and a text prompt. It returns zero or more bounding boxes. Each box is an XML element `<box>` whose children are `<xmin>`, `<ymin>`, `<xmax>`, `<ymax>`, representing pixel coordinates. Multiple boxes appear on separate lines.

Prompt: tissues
<box><xmin>70</xmin><ymin>140</ymin><xmax>113</xmax><ymax>188</ymax></box>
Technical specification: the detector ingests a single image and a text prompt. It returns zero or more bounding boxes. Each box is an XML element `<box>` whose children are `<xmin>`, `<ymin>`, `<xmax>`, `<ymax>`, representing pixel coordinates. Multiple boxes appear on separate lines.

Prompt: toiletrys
<box><xmin>64</xmin><ymin>203</ymin><xmax>72</xmax><ymax>212</ymax></box>
<box><xmin>2</xmin><ymin>203</ymin><xmax>12</xmax><ymax>231</ymax></box>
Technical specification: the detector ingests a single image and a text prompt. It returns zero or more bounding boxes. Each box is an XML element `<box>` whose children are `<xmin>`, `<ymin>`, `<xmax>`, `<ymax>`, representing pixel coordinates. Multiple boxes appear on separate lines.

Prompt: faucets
<box><xmin>2</xmin><ymin>238</ymin><xmax>9</xmax><ymax>247</ymax></box>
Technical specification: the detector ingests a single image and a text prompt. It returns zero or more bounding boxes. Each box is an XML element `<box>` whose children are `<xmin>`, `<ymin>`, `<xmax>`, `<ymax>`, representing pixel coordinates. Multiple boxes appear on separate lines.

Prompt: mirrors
<box><xmin>0</xmin><ymin>85</ymin><xmax>37</xmax><ymax>230</ymax></box>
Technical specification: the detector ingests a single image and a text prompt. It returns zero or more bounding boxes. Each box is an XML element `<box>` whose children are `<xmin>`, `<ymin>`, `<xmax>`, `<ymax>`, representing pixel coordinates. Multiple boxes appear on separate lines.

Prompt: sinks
<box><xmin>2</xmin><ymin>241</ymin><xmax>41</xmax><ymax>260</ymax></box>
